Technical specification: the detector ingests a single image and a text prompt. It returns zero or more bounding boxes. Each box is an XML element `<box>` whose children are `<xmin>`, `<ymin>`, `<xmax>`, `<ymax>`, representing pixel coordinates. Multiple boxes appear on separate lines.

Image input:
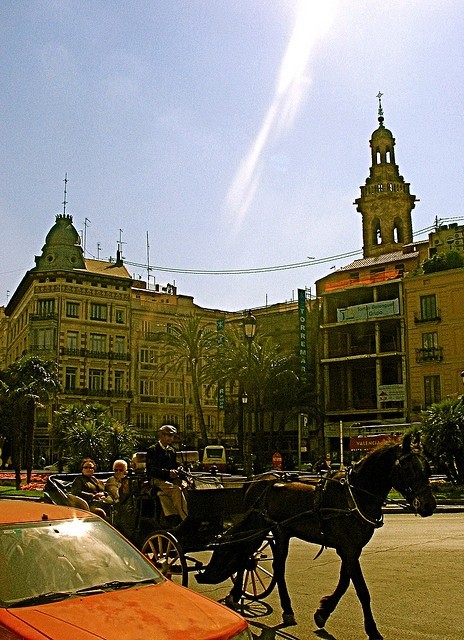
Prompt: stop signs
<box><xmin>272</xmin><ymin>452</ymin><xmax>282</xmax><ymax>466</ymax></box>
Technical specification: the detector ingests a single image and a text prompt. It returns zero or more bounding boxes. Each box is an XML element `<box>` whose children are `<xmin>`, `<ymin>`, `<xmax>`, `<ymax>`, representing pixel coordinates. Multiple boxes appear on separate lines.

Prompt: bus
<box><xmin>132</xmin><ymin>451</ymin><xmax>200</xmax><ymax>468</ymax></box>
<box><xmin>203</xmin><ymin>446</ymin><xmax>226</xmax><ymax>472</ymax></box>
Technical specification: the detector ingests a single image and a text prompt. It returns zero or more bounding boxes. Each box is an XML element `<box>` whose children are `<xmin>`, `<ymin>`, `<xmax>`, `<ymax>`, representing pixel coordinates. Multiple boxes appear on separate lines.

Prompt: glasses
<box><xmin>114</xmin><ymin>468</ymin><xmax>124</xmax><ymax>472</ymax></box>
<box><xmin>83</xmin><ymin>466</ymin><xmax>94</xmax><ymax>470</ymax></box>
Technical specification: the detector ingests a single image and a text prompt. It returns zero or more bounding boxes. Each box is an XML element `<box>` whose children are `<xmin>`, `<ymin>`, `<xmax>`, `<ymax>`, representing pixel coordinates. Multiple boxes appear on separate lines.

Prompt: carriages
<box><xmin>45</xmin><ymin>432</ymin><xmax>436</xmax><ymax>640</ymax></box>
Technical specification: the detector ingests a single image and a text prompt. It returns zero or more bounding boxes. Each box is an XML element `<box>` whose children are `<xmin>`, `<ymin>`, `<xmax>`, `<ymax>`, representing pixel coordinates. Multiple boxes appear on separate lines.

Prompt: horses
<box><xmin>226</xmin><ymin>432</ymin><xmax>436</xmax><ymax>640</ymax></box>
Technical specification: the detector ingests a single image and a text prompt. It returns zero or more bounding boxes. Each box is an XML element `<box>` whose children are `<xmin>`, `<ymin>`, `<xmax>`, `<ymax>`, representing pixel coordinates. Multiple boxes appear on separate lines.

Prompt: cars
<box><xmin>329</xmin><ymin>463</ymin><xmax>340</xmax><ymax>474</ymax></box>
<box><xmin>1</xmin><ymin>498</ymin><xmax>252</xmax><ymax>640</ymax></box>
<box><xmin>301</xmin><ymin>464</ymin><xmax>312</xmax><ymax>471</ymax></box>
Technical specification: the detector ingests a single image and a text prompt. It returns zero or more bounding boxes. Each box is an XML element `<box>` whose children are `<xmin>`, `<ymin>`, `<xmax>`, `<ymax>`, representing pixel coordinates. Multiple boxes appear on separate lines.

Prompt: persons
<box><xmin>146</xmin><ymin>425</ymin><xmax>190</xmax><ymax>521</ymax></box>
<box><xmin>71</xmin><ymin>458</ymin><xmax>105</xmax><ymax>510</ymax></box>
<box><xmin>103</xmin><ymin>459</ymin><xmax>133</xmax><ymax>503</ymax></box>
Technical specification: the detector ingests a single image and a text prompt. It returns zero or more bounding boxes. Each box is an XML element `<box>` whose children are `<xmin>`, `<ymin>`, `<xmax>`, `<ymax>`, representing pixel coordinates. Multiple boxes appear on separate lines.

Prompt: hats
<box><xmin>160</xmin><ymin>425</ymin><xmax>177</xmax><ymax>435</ymax></box>
